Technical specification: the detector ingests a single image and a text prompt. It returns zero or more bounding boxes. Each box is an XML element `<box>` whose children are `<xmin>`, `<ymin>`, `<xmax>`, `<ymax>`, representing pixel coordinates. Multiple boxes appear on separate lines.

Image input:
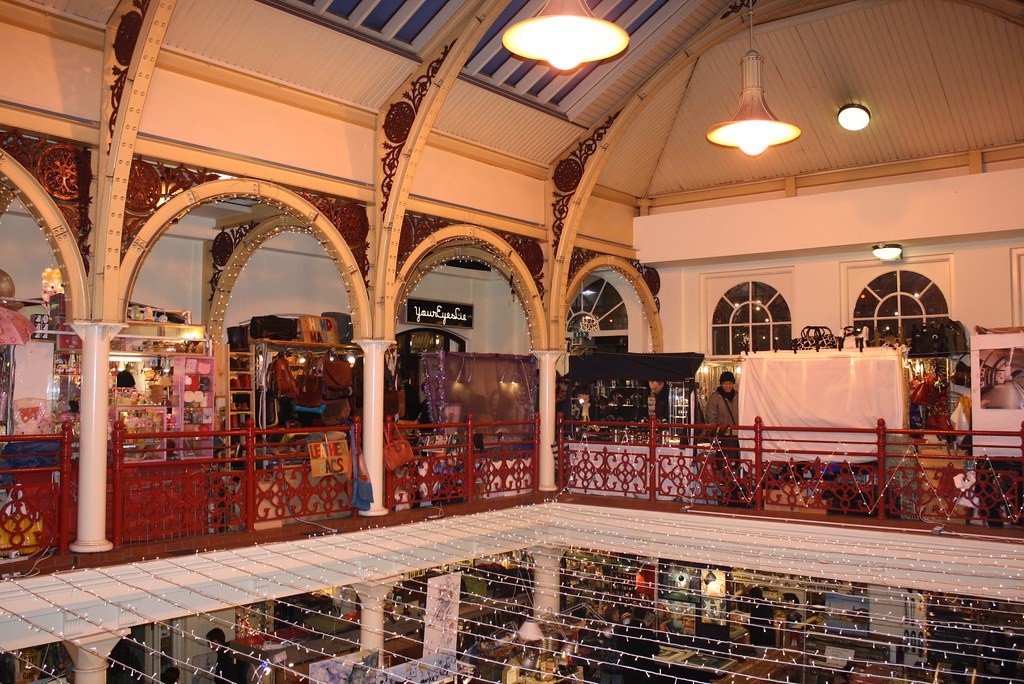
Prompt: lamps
<box><xmin>502</xmin><ymin>0</ymin><xmax>630</xmax><ymax>70</ymax></box>
<box><xmin>706</xmin><ymin>0</ymin><xmax>802</xmax><ymax>156</ymax></box>
<box><xmin>296</xmin><ymin>349</ymin><xmax>305</xmax><ymax>363</ymax></box>
<box><xmin>41</xmin><ymin>255</ymin><xmax>65</xmax><ymax>302</ymax></box>
<box><xmin>872</xmin><ymin>243</ymin><xmax>903</xmax><ymax>260</ymax></box>
<box><xmin>519</xmin><ymin>620</ymin><xmax>544</xmax><ymax>668</ymax></box>
<box><xmin>455</xmin><ymin>356</ymin><xmax>476</xmax><ymax>382</ymax></box>
<box><xmin>499</xmin><ymin>358</ymin><xmax>517</xmax><ymax>384</ymax></box>
<box><xmin>837</xmin><ymin>103</ymin><xmax>870</xmax><ymax>131</ymax></box>
<box><xmin>146</xmin><ymin>166</ymin><xmax>188</xmax><ymax>224</ymax></box>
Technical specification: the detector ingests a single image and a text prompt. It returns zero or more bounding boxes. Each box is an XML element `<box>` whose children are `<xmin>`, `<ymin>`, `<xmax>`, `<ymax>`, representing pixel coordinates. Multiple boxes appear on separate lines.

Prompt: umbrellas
<box><xmin>0</xmin><ymin>306</ymin><xmax>37</xmax><ymax>345</ymax></box>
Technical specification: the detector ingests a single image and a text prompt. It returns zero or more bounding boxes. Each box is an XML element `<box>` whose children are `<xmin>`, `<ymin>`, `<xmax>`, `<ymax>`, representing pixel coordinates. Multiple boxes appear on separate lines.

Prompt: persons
<box><xmin>649</xmin><ymin>380</ymin><xmax>670</xmax><ymax>432</ymax></box>
<box><xmin>206</xmin><ymin>627</ymin><xmax>252</xmax><ymax>684</ymax></box>
<box><xmin>410</xmin><ymin>377</ymin><xmax>446</xmax><ymax>508</ymax></box>
<box><xmin>600</xmin><ymin>607</ymin><xmax>660</xmax><ymax>684</ymax></box>
<box><xmin>750</xmin><ymin>587</ymin><xmax>777</xmax><ymax>648</ymax></box>
<box><xmin>705</xmin><ymin>371</ymin><xmax>738</xmax><ymax>507</ymax></box>
<box><xmin>572</xmin><ymin>384</ymin><xmax>600</xmax><ymax>441</ymax></box>
<box><xmin>160</xmin><ymin>667</ymin><xmax>180</xmax><ymax>684</ymax></box>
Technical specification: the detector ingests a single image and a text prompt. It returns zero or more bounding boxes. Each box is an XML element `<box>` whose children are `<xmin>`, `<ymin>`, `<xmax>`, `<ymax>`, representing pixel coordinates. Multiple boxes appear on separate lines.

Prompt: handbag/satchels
<box><xmin>384</xmin><ymin>387</ymin><xmax>405</xmax><ymax>417</ymax></box>
<box><xmin>383</xmin><ymin>423</ymin><xmax>414</xmax><ymax>471</ymax></box>
<box><xmin>256</xmin><ymin>348</ymin><xmax>354</xmax><ymax>429</ymax></box>
<box><xmin>789</xmin><ymin>317</ymin><xmax>972</xmax><ymax>468</ymax></box>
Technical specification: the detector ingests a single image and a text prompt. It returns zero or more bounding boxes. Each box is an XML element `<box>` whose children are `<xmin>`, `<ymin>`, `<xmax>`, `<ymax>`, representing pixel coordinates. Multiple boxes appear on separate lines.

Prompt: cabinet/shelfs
<box><xmin>213</xmin><ymin>344</ymin><xmax>254</xmax><ymax>485</ymax></box>
<box><xmin>594</xmin><ymin>387</ymin><xmax>648</xmax><ymax>407</ymax></box>
<box><xmin>52</xmin><ymin>320</ymin><xmax>214</xmax><ymax>464</ymax></box>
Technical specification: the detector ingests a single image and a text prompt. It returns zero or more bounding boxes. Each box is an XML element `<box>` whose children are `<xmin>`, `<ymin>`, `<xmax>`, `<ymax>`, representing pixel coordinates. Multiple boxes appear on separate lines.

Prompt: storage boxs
<box><xmin>763</xmin><ymin>484</ymin><xmax>827</xmax><ymax>514</ymax></box>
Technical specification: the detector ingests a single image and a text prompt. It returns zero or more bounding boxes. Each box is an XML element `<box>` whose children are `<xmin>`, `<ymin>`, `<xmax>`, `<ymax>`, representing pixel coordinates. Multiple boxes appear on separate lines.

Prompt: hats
<box><xmin>719</xmin><ymin>371</ymin><xmax>736</xmax><ymax>385</ymax></box>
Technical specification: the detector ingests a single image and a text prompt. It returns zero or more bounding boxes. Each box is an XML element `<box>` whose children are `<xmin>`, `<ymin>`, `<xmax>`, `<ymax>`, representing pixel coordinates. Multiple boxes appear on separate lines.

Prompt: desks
<box><xmin>234</xmin><ymin>593</ymin><xmax>533</xmax><ymax>684</ymax></box>
<box><xmin>713</xmin><ymin>649</ymin><xmax>804</xmax><ymax>684</ymax></box>
